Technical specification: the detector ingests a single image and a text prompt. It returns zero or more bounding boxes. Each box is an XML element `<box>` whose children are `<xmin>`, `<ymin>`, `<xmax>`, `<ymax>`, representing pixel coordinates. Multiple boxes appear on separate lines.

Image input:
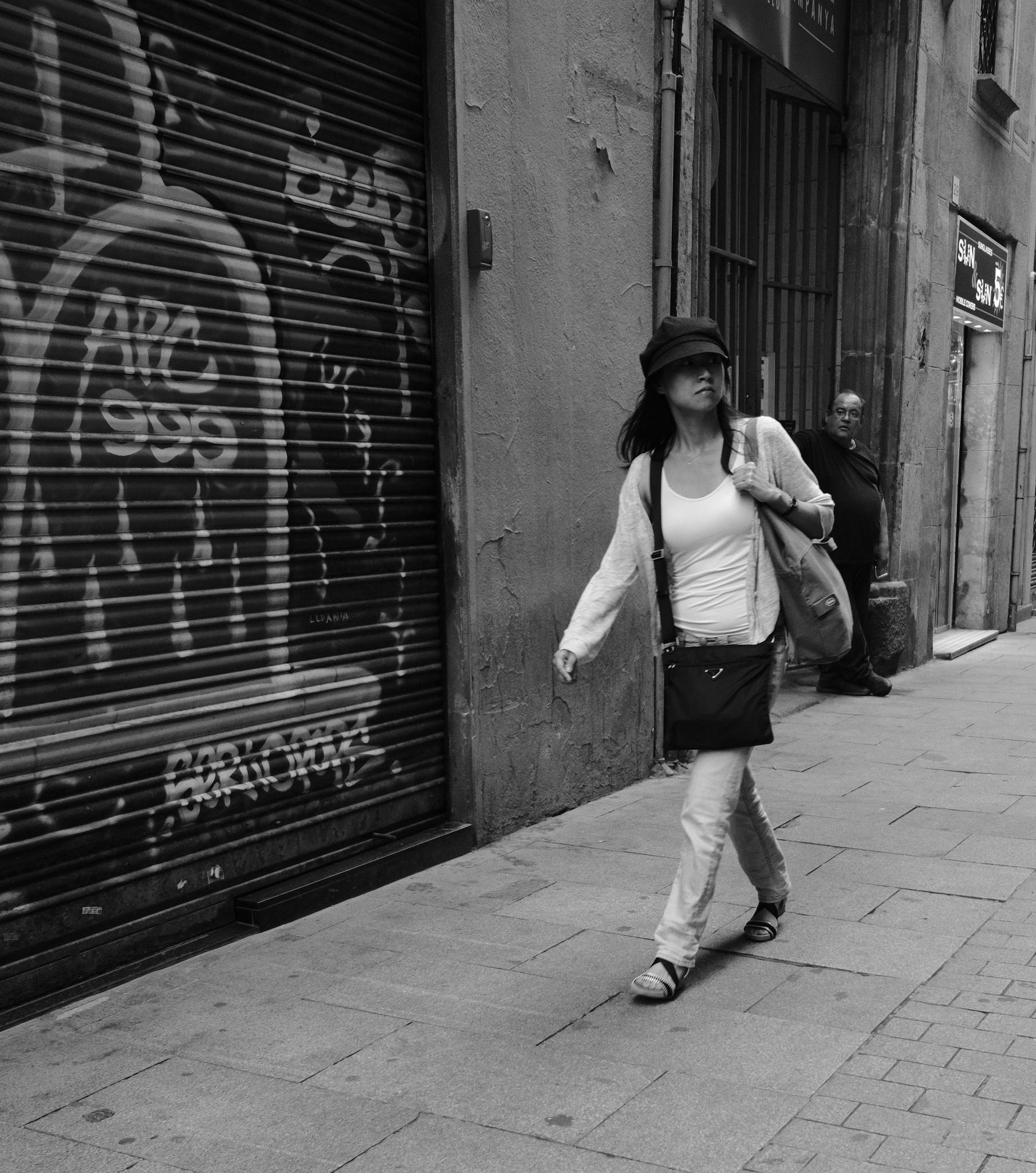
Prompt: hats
<box><xmin>640</xmin><ymin>314</ymin><xmax>728</xmax><ymax>375</ymax></box>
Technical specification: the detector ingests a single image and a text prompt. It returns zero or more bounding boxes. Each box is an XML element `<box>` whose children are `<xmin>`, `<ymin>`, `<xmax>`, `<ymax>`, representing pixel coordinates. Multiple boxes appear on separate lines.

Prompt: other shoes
<box><xmin>816</xmin><ymin>671</ymin><xmax>892</xmax><ymax>697</ymax></box>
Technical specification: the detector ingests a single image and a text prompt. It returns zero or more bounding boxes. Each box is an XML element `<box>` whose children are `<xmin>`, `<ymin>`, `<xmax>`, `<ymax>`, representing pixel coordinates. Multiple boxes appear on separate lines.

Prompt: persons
<box><xmin>788</xmin><ymin>390</ymin><xmax>892</xmax><ymax>697</ymax></box>
<box><xmin>549</xmin><ymin>314</ymin><xmax>835</xmax><ymax>999</ymax></box>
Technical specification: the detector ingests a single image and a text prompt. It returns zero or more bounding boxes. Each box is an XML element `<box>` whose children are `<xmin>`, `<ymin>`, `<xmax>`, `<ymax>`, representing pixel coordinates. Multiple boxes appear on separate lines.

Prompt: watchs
<box><xmin>777</xmin><ymin>494</ymin><xmax>798</xmax><ymax>518</ymax></box>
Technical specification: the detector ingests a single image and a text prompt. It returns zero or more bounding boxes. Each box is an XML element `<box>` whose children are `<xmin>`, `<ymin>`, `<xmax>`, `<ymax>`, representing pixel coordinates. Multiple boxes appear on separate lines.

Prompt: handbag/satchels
<box><xmin>661</xmin><ymin>642</ymin><xmax>774</xmax><ymax>753</ymax></box>
<box><xmin>743</xmin><ymin>416</ymin><xmax>854</xmax><ymax>666</ymax></box>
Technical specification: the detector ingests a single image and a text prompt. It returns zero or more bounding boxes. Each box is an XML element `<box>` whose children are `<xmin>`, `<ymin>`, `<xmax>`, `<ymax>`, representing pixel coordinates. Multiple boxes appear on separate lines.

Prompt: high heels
<box><xmin>631</xmin><ymin>958</ymin><xmax>690</xmax><ymax>999</ymax></box>
<box><xmin>743</xmin><ymin>897</ymin><xmax>786</xmax><ymax>940</ymax></box>
